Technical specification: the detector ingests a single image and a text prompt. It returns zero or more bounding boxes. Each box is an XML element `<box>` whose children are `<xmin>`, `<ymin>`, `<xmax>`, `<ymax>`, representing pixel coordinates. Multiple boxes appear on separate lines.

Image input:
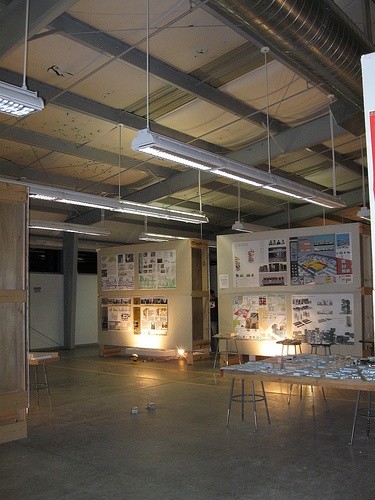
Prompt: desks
<box><xmin>311</xmin><ymin>343</ymin><xmax>332</xmax><ymax>355</ymax></box>
<box><xmin>277</xmin><ymin>339</ymin><xmax>302</xmax><ymax>356</ymax></box>
<box><xmin>220</xmin><ymin>354</ymin><xmax>375</xmax><ymax>446</ymax></box>
<box><xmin>212</xmin><ymin>333</ymin><xmax>241</xmax><ymax>369</ymax></box>
<box><xmin>28</xmin><ymin>357</ymin><xmax>60</xmax><ymax>413</ymax></box>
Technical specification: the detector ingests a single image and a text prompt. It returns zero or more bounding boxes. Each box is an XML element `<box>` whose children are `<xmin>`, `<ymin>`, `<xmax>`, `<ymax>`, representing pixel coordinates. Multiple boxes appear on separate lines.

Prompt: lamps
<box><xmin>0</xmin><ymin>123</ymin><xmax>371</xmax><ymax>248</ymax></box>
<box><xmin>0</xmin><ymin>0</ymin><xmax>44</xmax><ymax>118</ymax></box>
<box><xmin>131</xmin><ymin>0</ymin><xmax>347</xmax><ymax>208</ymax></box>
